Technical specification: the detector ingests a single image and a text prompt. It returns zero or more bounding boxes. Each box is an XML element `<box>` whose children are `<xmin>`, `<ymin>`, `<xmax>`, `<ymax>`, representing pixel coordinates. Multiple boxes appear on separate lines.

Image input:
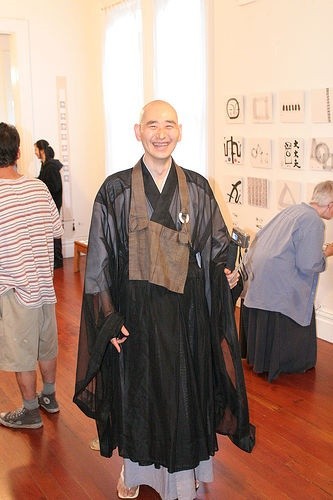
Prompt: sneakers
<box><xmin>38</xmin><ymin>391</ymin><xmax>59</xmax><ymax>413</ymax></box>
<box><xmin>0</xmin><ymin>407</ymin><xmax>43</xmax><ymax>429</ymax></box>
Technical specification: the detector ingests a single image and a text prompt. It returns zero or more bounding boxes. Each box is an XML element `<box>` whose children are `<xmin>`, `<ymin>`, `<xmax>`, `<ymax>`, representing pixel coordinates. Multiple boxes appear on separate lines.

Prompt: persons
<box><xmin>73</xmin><ymin>100</ymin><xmax>256</xmax><ymax>500</ymax></box>
<box><xmin>0</xmin><ymin>121</ymin><xmax>64</xmax><ymax>430</ymax></box>
<box><xmin>34</xmin><ymin>139</ymin><xmax>63</xmax><ymax>270</ymax></box>
<box><xmin>239</xmin><ymin>181</ymin><xmax>333</xmax><ymax>382</ymax></box>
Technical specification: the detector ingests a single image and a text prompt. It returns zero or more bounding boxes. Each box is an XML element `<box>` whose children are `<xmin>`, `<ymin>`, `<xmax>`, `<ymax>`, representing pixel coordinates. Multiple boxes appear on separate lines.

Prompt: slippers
<box><xmin>118</xmin><ymin>464</ymin><xmax>139</xmax><ymax>498</ymax></box>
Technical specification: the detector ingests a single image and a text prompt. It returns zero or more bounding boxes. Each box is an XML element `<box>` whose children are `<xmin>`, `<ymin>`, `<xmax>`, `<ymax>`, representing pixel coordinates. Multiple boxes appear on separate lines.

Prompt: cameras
<box><xmin>231</xmin><ymin>228</ymin><xmax>250</xmax><ymax>249</ymax></box>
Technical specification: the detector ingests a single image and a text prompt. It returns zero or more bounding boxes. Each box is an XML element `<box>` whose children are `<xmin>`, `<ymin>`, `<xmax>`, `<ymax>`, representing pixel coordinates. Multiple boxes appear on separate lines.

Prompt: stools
<box><xmin>73</xmin><ymin>239</ymin><xmax>88</xmax><ymax>273</ymax></box>
